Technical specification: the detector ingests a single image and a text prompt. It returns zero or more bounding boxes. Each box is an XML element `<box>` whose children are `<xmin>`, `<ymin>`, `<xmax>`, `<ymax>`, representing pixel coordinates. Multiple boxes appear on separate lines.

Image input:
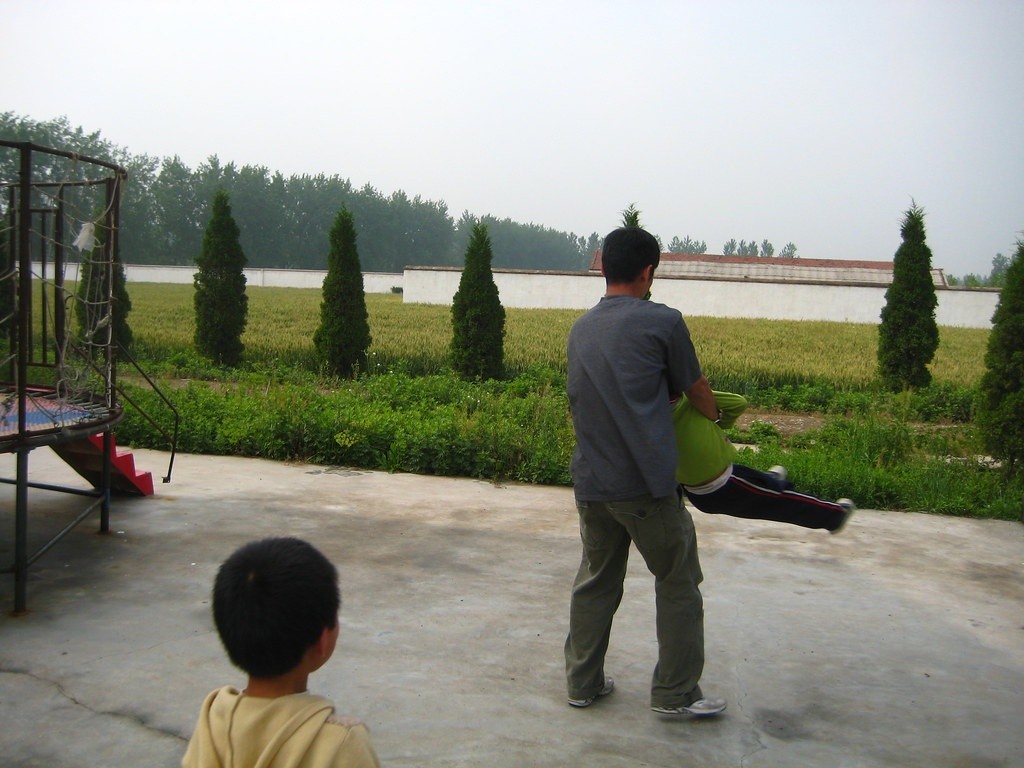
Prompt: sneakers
<box><xmin>650</xmin><ymin>699</ymin><xmax>727</xmax><ymax>713</ymax></box>
<box><xmin>568</xmin><ymin>675</ymin><xmax>614</xmax><ymax>706</ymax></box>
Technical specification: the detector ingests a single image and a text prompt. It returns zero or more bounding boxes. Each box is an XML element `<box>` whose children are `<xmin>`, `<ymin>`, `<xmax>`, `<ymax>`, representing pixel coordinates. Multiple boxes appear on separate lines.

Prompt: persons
<box><xmin>181</xmin><ymin>536</ymin><xmax>383</xmax><ymax>768</ymax></box>
<box><xmin>658</xmin><ymin>388</ymin><xmax>857</xmax><ymax>537</ymax></box>
<box><xmin>561</xmin><ymin>224</ymin><xmax>731</xmax><ymax>723</ymax></box>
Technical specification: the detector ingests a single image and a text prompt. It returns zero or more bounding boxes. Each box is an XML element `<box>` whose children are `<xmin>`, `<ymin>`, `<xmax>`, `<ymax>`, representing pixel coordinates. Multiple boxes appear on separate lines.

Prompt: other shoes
<box><xmin>829</xmin><ymin>498</ymin><xmax>854</xmax><ymax>534</ymax></box>
<box><xmin>767</xmin><ymin>464</ymin><xmax>787</xmax><ymax>481</ymax></box>
